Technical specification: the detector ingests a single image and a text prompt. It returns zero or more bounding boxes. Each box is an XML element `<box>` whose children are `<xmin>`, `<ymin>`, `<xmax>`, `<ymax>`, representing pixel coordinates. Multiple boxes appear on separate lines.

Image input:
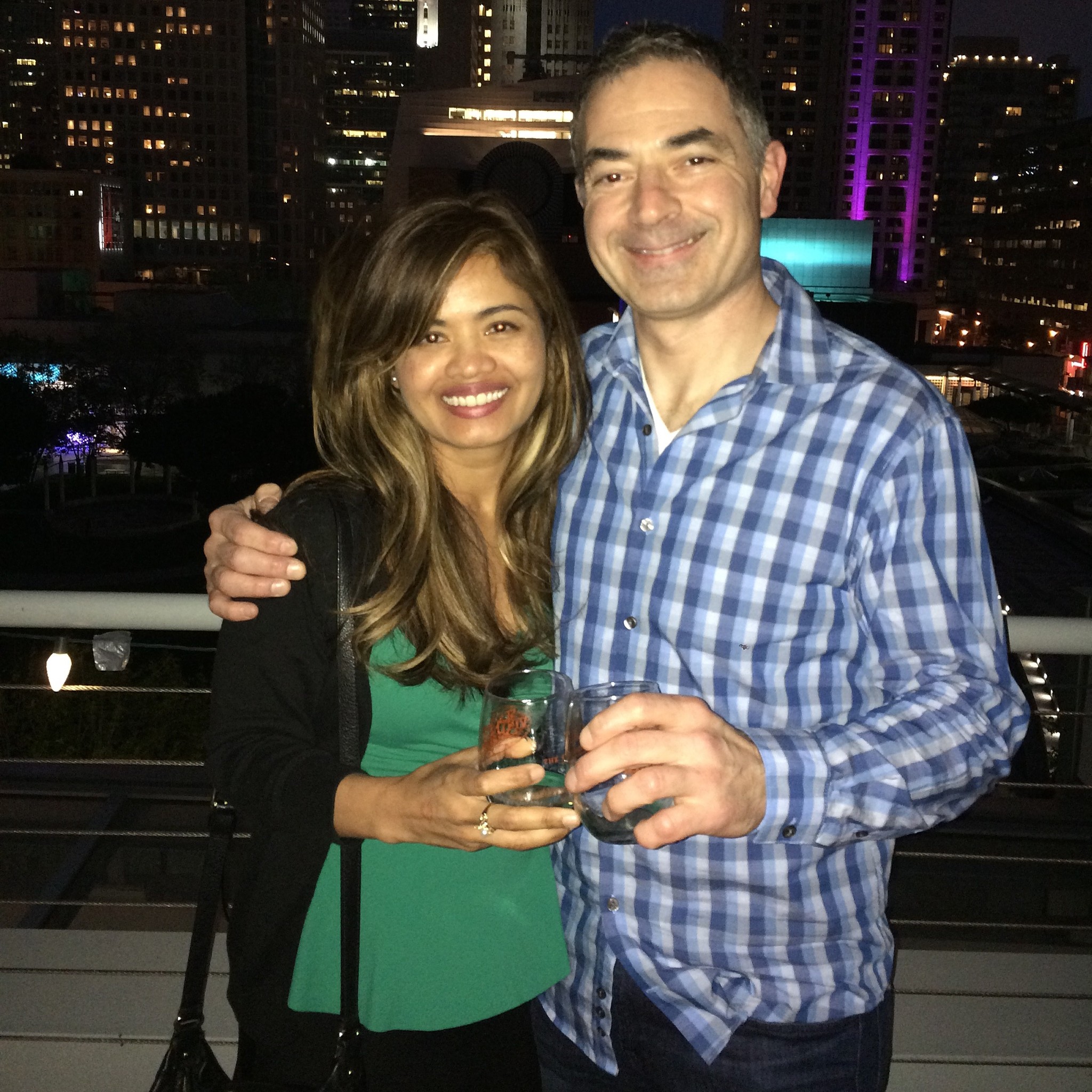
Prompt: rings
<box><xmin>476</xmin><ymin>805</ymin><xmax>499</xmax><ymax>838</ymax></box>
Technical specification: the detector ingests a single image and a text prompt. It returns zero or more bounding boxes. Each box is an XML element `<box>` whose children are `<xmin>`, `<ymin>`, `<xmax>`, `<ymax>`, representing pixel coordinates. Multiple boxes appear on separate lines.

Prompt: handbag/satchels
<box><xmin>149</xmin><ymin>1009</ymin><xmax>369</xmax><ymax>1092</ymax></box>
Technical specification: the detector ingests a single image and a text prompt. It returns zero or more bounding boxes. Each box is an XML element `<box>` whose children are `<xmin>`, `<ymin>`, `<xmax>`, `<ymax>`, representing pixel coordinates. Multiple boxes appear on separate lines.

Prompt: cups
<box><xmin>563</xmin><ymin>680</ymin><xmax>674</xmax><ymax>845</ymax></box>
<box><xmin>478</xmin><ymin>669</ymin><xmax>583</xmax><ymax>809</ymax></box>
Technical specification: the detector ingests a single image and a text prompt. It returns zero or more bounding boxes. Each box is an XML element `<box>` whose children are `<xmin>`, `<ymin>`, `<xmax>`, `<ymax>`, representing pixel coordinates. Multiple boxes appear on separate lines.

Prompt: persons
<box><xmin>204</xmin><ymin>197</ymin><xmax>575</xmax><ymax>1092</ymax></box>
<box><xmin>196</xmin><ymin>15</ymin><xmax>1032</xmax><ymax>1092</ymax></box>
